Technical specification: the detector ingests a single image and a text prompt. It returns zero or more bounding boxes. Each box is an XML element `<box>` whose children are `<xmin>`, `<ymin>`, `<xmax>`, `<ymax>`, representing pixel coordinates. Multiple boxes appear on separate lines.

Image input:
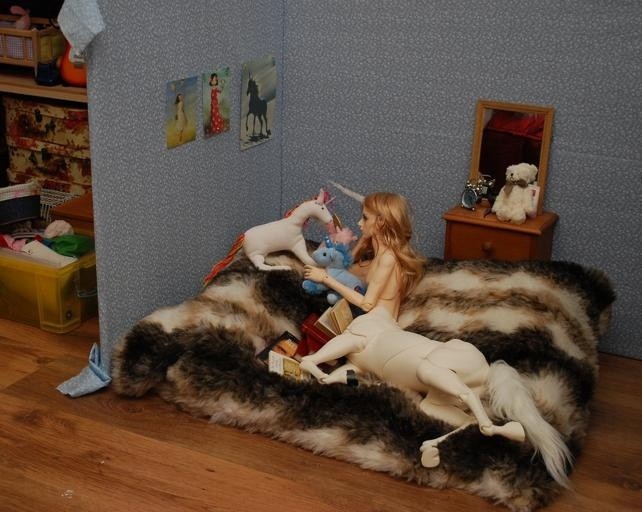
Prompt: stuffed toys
<box><xmin>201</xmin><ymin>180</ymin><xmax>578</xmax><ymax>493</ymax></box>
<box><xmin>489</xmin><ymin>161</ymin><xmax>540</xmax><ymax>225</ymax></box>
<box><xmin>203</xmin><ymin>188</ymin><xmax>366</xmax><ymax>305</ymax></box>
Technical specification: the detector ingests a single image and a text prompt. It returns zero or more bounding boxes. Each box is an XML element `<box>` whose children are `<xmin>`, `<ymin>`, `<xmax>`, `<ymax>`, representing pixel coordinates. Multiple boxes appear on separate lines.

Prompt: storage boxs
<box><xmin>0</xmin><ymin>223</ymin><xmax>97</xmax><ymax>335</ymax></box>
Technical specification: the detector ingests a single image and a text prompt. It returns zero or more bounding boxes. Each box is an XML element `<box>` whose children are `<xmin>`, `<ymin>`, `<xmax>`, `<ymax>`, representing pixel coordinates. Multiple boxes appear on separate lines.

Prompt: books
<box><xmin>255</xmin><ymin>299</ymin><xmax>354</xmax><ymax>384</ymax></box>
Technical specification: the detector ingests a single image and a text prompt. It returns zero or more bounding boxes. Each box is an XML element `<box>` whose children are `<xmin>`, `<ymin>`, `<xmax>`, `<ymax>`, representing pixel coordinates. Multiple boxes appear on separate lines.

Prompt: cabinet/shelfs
<box><xmin>0</xmin><ymin>1</ymin><xmax>93</xmax><ymax>198</ymax></box>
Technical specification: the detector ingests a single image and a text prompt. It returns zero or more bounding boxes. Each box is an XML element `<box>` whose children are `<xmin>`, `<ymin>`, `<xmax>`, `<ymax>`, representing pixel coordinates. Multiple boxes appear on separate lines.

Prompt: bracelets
<box><xmin>322</xmin><ymin>274</ymin><xmax>328</xmax><ymax>283</ymax></box>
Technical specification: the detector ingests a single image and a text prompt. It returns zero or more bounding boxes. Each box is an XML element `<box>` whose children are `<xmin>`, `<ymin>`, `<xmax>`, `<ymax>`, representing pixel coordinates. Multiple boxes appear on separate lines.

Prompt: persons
<box><xmin>302</xmin><ymin>192</ymin><xmax>426</xmax><ymax>320</ymax></box>
<box><xmin>206</xmin><ymin>73</ymin><xmax>226</xmax><ymax>134</ymax></box>
<box><xmin>174</xmin><ymin>93</ymin><xmax>190</xmax><ymax>145</ymax></box>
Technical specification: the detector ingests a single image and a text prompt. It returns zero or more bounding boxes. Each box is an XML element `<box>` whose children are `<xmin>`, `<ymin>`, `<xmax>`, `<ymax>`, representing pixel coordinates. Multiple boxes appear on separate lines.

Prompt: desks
<box><xmin>48</xmin><ymin>192</ymin><xmax>94</xmax><ymax>239</ymax></box>
